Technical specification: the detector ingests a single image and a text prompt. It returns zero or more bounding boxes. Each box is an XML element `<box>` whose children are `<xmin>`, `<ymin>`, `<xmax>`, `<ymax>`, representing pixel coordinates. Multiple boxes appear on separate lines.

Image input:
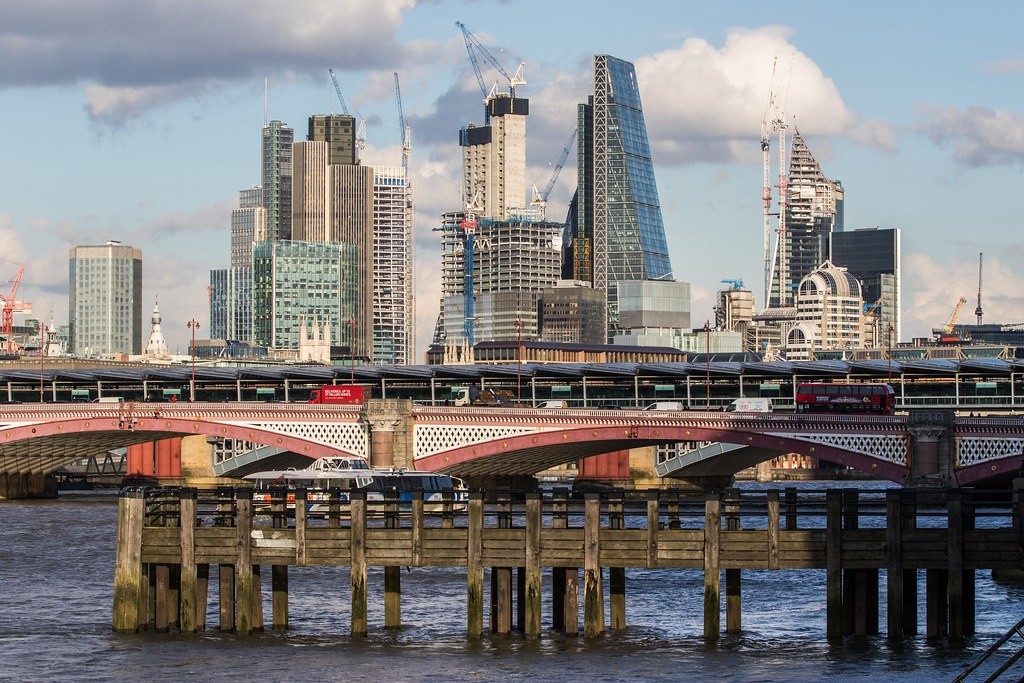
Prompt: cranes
<box><xmin>935</xmin><ymin>297</ymin><xmax>967</xmax><ymax>338</ymax></box>
<box><xmin>0</xmin><ymin>261</ymin><xmax>26</xmax><ymax>357</ymax></box>
<box><xmin>716</xmin><ymin>48</ymin><xmax>890</xmax><ymax>351</ymax></box>
<box><xmin>322</xmin><ymin>21</ymin><xmax>578</xmax><ymax>222</ymax></box>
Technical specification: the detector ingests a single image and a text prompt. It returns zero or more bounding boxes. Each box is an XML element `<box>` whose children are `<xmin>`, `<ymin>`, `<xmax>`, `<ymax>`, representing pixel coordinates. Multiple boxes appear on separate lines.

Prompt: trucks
<box><xmin>454</xmin><ymin>388</ymin><xmax>515</xmax><ymax>406</ymax></box>
<box><xmin>308</xmin><ymin>383</ymin><xmax>364</xmax><ymax>406</ymax></box>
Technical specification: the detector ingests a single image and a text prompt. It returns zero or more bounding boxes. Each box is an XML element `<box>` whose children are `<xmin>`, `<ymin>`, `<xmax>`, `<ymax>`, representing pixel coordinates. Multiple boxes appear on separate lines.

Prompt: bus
<box><xmin>792</xmin><ymin>380</ymin><xmax>899</xmax><ymax>416</ymax></box>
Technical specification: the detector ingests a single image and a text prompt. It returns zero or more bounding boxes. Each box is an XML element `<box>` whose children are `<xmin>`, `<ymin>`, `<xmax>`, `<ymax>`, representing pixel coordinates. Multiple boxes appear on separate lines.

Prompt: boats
<box><xmin>244</xmin><ymin>454</ymin><xmax>471</xmax><ymax>518</ymax></box>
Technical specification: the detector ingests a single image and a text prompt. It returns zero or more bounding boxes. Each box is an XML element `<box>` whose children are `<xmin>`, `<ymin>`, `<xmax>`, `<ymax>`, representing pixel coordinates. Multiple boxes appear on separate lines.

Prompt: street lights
<box><xmin>185</xmin><ymin>316</ymin><xmax>202</xmax><ymax>401</ymax></box>
